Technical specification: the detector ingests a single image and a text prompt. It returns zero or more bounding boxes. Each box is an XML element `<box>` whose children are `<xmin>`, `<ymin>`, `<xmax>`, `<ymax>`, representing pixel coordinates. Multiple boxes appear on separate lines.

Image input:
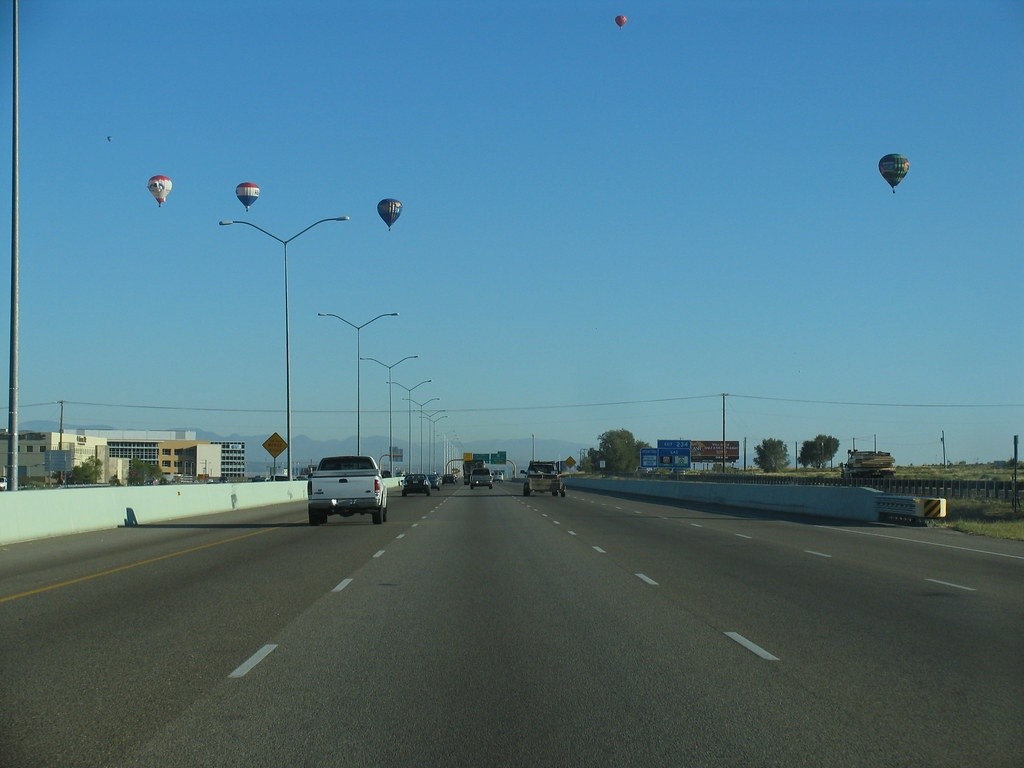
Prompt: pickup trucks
<box><xmin>307</xmin><ymin>455</ymin><xmax>387</xmax><ymax>525</ymax></box>
<box><xmin>521</xmin><ymin>461</ymin><xmax>567</xmax><ymax>498</ymax></box>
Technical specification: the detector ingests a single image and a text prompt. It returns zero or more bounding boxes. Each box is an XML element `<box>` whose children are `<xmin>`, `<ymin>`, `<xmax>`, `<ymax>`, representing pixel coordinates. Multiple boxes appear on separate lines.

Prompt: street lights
<box><xmin>318</xmin><ymin>313</ymin><xmax>400</xmax><ymax>468</ymax></box>
<box><xmin>402</xmin><ymin>398</ymin><xmax>448</xmax><ymax>472</ymax></box>
<box><xmin>360</xmin><ymin>356</ymin><xmax>418</xmax><ymax>478</ymax></box>
<box><xmin>532</xmin><ymin>434</ymin><xmax>534</xmax><ymax>461</ymax></box>
<box><xmin>219</xmin><ymin>216</ymin><xmax>352</xmax><ymax>481</ymax></box>
<box><xmin>386</xmin><ymin>380</ymin><xmax>431</xmax><ymax>474</ymax></box>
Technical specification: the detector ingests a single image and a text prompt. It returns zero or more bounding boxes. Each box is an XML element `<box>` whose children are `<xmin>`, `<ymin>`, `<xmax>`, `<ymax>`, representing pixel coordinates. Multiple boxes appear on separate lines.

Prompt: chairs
<box><xmin>359</xmin><ymin>465</ymin><xmax>370</xmax><ymax>469</ymax></box>
<box><xmin>325</xmin><ymin>465</ymin><xmax>334</xmax><ymax>470</ymax></box>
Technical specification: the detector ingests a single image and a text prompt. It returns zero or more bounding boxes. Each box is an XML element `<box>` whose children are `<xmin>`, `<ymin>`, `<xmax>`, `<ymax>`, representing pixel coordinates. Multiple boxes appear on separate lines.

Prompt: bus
<box><xmin>463</xmin><ymin>461</ymin><xmax>485</xmax><ymax>484</ymax></box>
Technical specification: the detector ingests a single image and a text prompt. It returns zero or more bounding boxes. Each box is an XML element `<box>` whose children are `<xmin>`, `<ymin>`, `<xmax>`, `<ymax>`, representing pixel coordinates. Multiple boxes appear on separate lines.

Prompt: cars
<box><xmin>427</xmin><ymin>473</ymin><xmax>458</xmax><ymax>491</ymax></box>
<box><xmin>218</xmin><ymin>477</ymin><xmax>229</xmax><ymax>483</ymax></box>
<box><xmin>208</xmin><ymin>479</ymin><xmax>213</xmax><ymax>483</ymax></box>
<box><xmin>401</xmin><ymin>474</ymin><xmax>430</xmax><ymax>497</ymax></box>
<box><xmin>0</xmin><ymin>476</ymin><xmax>7</xmax><ymax>490</ymax></box>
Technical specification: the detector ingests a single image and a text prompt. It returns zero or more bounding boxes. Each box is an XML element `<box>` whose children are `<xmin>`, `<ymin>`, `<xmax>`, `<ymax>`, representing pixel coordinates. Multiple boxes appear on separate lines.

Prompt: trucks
<box><xmin>470</xmin><ymin>468</ymin><xmax>504</xmax><ymax>489</ymax></box>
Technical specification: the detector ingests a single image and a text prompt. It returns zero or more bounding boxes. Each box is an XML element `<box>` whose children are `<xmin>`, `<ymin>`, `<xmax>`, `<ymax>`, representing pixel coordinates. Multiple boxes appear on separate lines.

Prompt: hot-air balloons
<box><xmin>615</xmin><ymin>15</ymin><xmax>625</xmax><ymax>30</ymax></box>
<box><xmin>147</xmin><ymin>176</ymin><xmax>172</xmax><ymax>208</ymax></box>
<box><xmin>377</xmin><ymin>199</ymin><xmax>403</xmax><ymax>231</ymax></box>
<box><xmin>878</xmin><ymin>154</ymin><xmax>910</xmax><ymax>194</ymax></box>
<box><xmin>236</xmin><ymin>182</ymin><xmax>260</xmax><ymax>212</ymax></box>
